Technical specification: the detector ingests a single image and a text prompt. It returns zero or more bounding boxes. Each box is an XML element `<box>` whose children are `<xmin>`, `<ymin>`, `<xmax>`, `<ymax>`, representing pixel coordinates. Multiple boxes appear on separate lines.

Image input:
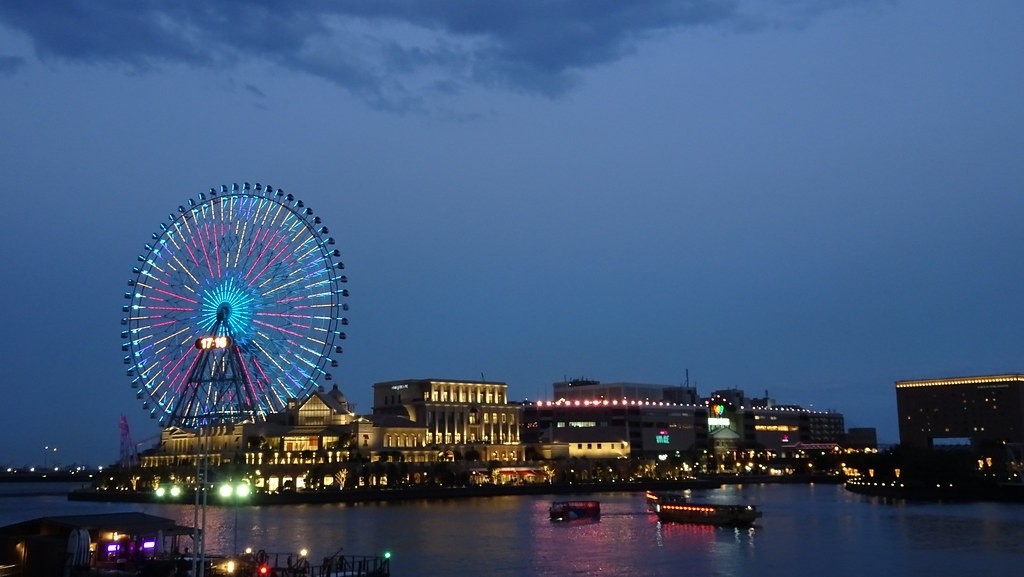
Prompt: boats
<box><xmin>646</xmin><ymin>489</ymin><xmax>759</xmax><ymax>525</ymax></box>
<box><xmin>548</xmin><ymin>499</ymin><xmax>600</xmax><ymax>521</ymax></box>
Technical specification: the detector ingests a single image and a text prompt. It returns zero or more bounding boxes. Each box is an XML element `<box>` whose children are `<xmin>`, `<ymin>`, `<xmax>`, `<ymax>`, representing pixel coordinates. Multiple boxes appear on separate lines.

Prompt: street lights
<box><xmin>220</xmin><ymin>484</ymin><xmax>250</xmax><ymax>555</ymax></box>
